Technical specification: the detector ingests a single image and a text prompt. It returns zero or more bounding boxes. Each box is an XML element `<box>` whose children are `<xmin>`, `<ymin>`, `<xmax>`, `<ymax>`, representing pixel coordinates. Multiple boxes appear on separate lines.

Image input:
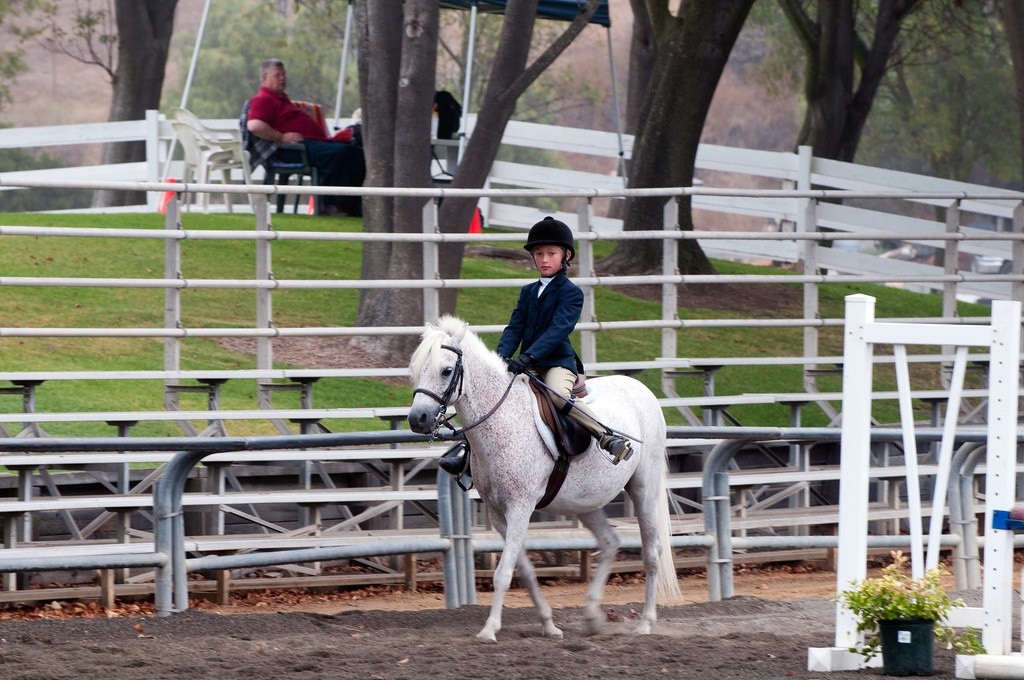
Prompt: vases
<box><xmin>877</xmin><ymin>617</ymin><xmax>934</xmax><ymax>676</ymax></box>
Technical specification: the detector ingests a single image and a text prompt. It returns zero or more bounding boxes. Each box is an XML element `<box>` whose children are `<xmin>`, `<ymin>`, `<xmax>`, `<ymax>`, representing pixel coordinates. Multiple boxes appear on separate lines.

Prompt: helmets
<box><xmin>523</xmin><ymin>217</ymin><xmax>575</xmax><ymax>262</ymax></box>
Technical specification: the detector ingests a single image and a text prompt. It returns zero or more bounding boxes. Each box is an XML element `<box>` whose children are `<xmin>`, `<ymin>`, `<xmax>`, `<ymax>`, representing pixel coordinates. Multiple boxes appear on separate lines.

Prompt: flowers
<box><xmin>831</xmin><ymin>550</ymin><xmax>989</xmax><ymax>662</ymax></box>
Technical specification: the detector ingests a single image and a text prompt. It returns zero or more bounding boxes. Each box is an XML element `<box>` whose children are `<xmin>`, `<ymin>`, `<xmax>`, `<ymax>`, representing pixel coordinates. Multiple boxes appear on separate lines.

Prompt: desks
<box><xmin>304</xmin><ymin>139</ymin><xmax>363</xmax><ymax>216</ymax></box>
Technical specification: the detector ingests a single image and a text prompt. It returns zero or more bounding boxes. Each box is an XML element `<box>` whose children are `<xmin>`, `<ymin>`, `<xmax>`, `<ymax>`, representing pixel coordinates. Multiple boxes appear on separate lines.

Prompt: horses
<box><xmin>406</xmin><ymin>313</ymin><xmax>683</xmax><ymax>644</ymax></box>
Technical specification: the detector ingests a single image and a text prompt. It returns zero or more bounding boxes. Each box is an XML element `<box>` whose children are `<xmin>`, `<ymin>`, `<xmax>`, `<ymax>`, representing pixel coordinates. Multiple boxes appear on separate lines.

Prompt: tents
<box><xmin>161</xmin><ymin>0</ymin><xmax>627</xmax><ymax>190</ymax></box>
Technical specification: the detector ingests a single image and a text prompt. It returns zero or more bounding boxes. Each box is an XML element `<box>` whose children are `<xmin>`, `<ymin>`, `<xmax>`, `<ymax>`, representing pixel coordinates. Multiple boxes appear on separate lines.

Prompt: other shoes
<box><xmin>605</xmin><ymin>439</ymin><xmax>634</xmax><ymax>461</ymax></box>
<box><xmin>438</xmin><ymin>455</ymin><xmax>470</xmax><ymax>476</ymax></box>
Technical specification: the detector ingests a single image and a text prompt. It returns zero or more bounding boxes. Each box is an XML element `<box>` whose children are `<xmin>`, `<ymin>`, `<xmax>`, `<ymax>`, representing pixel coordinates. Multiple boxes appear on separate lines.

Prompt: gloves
<box><xmin>507</xmin><ymin>354</ymin><xmax>531</xmax><ymax>374</ymax></box>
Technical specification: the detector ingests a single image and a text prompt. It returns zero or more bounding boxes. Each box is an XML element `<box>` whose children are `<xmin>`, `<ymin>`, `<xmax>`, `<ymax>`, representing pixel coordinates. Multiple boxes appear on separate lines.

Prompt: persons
<box><xmin>438</xmin><ymin>217</ymin><xmax>635</xmax><ymax>478</ymax></box>
<box><xmin>246</xmin><ymin>58</ymin><xmax>354</xmax><ymax>216</ymax></box>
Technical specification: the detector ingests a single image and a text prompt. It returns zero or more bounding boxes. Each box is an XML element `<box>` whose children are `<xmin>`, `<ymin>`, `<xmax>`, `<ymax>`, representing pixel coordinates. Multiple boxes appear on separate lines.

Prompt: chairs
<box><xmin>243</xmin><ymin>100</ymin><xmax>321</xmax><ymax>217</ymax></box>
<box><xmin>169</xmin><ymin>106</ymin><xmax>255</xmax><ymax>216</ymax></box>
